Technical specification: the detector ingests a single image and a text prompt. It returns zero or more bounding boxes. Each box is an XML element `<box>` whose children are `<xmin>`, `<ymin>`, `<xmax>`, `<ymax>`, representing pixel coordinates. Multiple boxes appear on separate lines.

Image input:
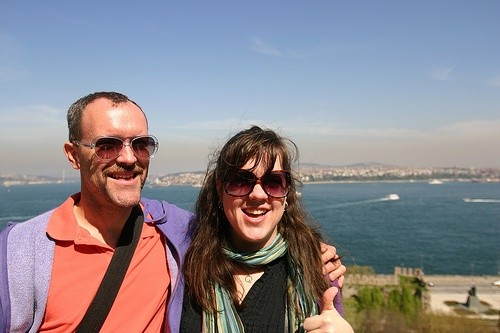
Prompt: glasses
<box><xmin>221</xmin><ymin>168</ymin><xmax>292</xmax><ymax>198</ymax></box>
<box><xmin>73</xmin><ymin>135</ymin><xmax>159</xmax><ymax>162</ymax></box>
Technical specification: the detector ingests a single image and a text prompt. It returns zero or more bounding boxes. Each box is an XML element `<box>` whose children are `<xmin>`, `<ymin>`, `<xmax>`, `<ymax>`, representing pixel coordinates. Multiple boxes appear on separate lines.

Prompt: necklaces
<box><xmin>242</xmin><ymin>268</ymin><xmax>254</xmax><ymax>283</ymax></box>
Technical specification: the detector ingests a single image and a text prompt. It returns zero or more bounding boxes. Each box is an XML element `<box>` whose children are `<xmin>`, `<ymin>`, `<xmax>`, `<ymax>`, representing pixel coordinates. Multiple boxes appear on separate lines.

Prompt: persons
<box><xmin>179</xmin><ymin>126</ymin><xmax>354</xmax><ymax>333</ymax></box>
<box><xmin>0</xmin><ymin>91</ymin><xmax>347</xmax><ymax>333</ymax></box>
